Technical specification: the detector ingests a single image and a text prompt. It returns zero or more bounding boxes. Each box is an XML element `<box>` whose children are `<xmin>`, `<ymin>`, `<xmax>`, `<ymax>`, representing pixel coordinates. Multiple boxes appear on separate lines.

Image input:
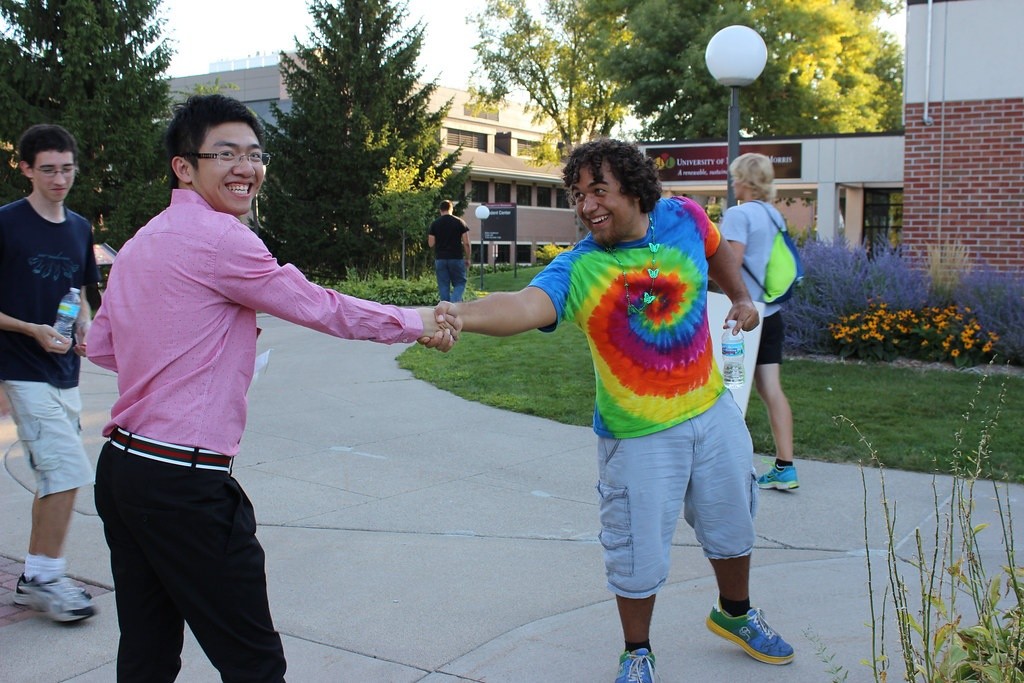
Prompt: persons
<box><xmin>417</xmin><ymin>138</ymin><xmax>794</xmax><ymax>683</ymax></box>
<box><xmin>428</xmin><ymin>200</ymin><xmax>470</xmax><ymax>302</ymax></box>
<box><xmin>85</xmin><ymin>95</ymin><xmax>461</xmax><ymax>683</ymax></box>
<box><xmin>0</xmin><ymin>125</ymin><xmax>102</xmax><ymax>622</ymax></box>
<box><xmin>708</xmin><ymin>152</ymin><xmax>799</xmax><ymax>490</ymax></box>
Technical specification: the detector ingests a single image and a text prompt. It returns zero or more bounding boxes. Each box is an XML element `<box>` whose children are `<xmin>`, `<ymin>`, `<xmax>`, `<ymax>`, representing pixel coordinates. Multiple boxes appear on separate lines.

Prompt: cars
<box><xmin>93</xmin><ymin>224</ymin><xmax>108</xmax><ymax>232</ymax></box>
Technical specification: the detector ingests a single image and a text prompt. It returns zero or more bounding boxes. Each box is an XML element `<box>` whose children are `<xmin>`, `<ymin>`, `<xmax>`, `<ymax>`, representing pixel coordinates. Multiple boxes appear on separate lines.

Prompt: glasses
<box><xmin>30</xmin><ymin>166</ymin><xmax>77</xmax><ymax>177</ymax></box>
<box><xmin>180</xmin><ymin>150</ymin><xmax>271</xmax><ymax>167</ymax></box>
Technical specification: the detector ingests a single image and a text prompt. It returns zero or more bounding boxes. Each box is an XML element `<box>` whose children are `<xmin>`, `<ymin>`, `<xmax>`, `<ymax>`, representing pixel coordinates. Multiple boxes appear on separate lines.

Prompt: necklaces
<box><xmin>604</xmin><ymin>212</ymin><xmax>660</xmax><ymax>317</ymax></box>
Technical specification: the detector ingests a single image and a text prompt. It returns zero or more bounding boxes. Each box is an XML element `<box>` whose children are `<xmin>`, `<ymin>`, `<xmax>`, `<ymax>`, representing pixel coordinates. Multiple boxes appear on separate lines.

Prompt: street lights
<box><xmin>252</xmin><ymin>166</ymin><xmax>266</xmax><ymax>237</ymax></box>
<box><xmin>705</xmin><ymin>25</ymin><xmax>768</xmax><ymax>209</ymax></box>
<box><xmin>475</xmin><ymin>205</ymin><xmax>489</xmax><ymax>292</ymax></box>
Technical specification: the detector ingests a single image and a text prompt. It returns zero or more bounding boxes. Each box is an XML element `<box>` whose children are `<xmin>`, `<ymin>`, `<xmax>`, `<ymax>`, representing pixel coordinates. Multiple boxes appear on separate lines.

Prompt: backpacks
<box><xmin>740</xmin><ymin>199</ymin><xmax>806</xmax><ymax>305</ymax></box>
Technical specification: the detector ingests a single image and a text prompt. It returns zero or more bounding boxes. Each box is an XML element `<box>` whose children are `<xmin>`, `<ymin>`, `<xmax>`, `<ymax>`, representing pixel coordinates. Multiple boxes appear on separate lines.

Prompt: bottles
<box><xmin>722</xmin><ymin>320</ymin><xmax>746</xmax><ymax>388</ymax></box>
<box><xmin>52</xmin><ymin>287</ymin><xmax>82</xmax><ymax>344</ymax></box>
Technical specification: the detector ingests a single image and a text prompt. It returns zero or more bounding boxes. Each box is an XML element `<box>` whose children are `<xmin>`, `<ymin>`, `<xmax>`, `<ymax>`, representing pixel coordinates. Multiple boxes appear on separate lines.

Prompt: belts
<box><xmin>108</xmin><ymin>426</ymin><xmax>236</xmax><ymax>472</ymax></box>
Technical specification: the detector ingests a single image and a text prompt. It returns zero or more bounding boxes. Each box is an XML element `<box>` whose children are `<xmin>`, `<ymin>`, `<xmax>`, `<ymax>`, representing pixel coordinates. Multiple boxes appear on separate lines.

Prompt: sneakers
<box><xmin>707</xmin><ymin>601</ymin><xmax>795</xmax><ymax>666</ymax></box>
<box><xmin>616</xmin><ymin>647</ymin><xmax>656</xmax><ymax>683</ymax></box>
<box><xmin>14</xmin><ymin>574</ymin><xmax>100</xmax><ymax>626</ymax></box>
<box><xmin>756</xmin><ymin>466</ymin><xmax>800</xmax><ymax>492</ymax></box>
